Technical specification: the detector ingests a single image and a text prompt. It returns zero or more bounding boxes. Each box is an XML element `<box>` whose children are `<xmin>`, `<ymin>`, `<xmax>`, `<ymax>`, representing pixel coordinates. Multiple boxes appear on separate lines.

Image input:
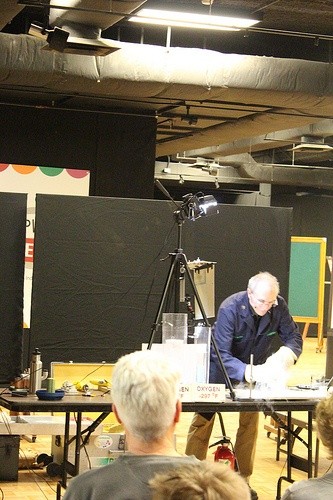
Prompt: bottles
<box><xmin>29</xmin><ymin>347</ymin><xmax>42</xmax><ymax>394</ymax></box>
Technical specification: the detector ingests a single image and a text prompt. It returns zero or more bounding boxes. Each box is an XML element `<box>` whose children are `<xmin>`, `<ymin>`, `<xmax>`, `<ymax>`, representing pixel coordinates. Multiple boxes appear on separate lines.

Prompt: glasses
<box><xmin>248</xmin><ymin>286</ymin><xmax>278</xmax><ymax>307</ymax></box>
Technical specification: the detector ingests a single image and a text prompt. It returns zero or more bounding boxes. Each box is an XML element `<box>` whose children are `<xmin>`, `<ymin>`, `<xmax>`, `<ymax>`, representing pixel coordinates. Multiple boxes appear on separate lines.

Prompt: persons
<box><xmin>62</xmin><ymin>350</ymin><xmax>253</xmax><ymax>500</ymax></box>
<box><xmin>281</xmin><ymin>392</ymin><xmax>333</xmax><ymax>500</ymax></box>
<box><xmin>185</xmin><ymin>271</ymin><xmax>302</xmax><ymax>500</ymax></box>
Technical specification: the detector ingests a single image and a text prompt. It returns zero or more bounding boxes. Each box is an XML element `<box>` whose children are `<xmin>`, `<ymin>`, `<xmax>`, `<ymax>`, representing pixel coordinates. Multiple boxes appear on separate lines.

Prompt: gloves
<box><xmin>245</xmin><ymin>364</ymin><xmax>278</xmax><ymax>385</ymax></box>
<box><xmin>266</xmin><ymin>346</ymin><xmax>297</xmax><ymax>369</ymax></box>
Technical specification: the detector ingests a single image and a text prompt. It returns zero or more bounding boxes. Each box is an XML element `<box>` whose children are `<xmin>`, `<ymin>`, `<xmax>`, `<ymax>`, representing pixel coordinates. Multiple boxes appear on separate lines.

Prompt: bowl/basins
<box><xmin>36</xmin><ymin>390</ymin><xmax>65</xmax><ymax>401</ymax></box>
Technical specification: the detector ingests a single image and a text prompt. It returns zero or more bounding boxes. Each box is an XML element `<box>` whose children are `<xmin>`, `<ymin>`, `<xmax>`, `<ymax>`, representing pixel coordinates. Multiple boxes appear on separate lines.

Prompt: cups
<box><xmin>311</xmin><ymin>374</ymin><xmax>326</xmax><ymax>386</ymax></box>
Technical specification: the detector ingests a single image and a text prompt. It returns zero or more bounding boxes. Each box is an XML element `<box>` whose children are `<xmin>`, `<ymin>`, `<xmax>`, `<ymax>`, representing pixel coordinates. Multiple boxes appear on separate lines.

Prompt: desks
<box><xmin>0</xmin><ymin>386</ymin><xmax>327</xmax><ymax>500</ymax></box>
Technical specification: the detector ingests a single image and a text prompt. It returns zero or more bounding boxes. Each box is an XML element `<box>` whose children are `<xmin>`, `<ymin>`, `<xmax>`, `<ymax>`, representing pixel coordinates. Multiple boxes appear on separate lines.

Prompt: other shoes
<box><xmin>247</xmin><ymin>483</ymin><xmax>258</xmax><ymax>500</ymax></box>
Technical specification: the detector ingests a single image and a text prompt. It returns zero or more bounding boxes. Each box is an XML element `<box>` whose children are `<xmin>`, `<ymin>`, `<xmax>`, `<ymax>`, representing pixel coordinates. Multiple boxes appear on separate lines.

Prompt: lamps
<box><xmin>285</xmin><ymin>135</ymin><xmax>333</xmax><ymax>153</ymax></box>
<box><xmin>26</xmin><ymin>20</ymin><xmax>121</xmax><ymax>58</ymax></box>
<box><xmin>147</xmin><ymin>179</ymin><xmax>236</xmax><ymax>400</ymax></box>
<box><xmin>126</xmin><ymin>2</ymin><xmax>263</xmax><ymax>32</ymax></box>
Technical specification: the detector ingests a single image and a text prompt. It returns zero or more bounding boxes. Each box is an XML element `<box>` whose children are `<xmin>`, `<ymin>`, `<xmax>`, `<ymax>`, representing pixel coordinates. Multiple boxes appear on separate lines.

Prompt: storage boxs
<box><xmin>51</xmin><ymin>430</ymin><xmax>128</xmax><ymax>478</ymax></box>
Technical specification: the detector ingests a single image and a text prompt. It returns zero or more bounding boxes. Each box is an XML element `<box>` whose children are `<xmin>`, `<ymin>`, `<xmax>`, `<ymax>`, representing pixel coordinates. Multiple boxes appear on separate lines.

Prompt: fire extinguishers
<box><xmin>207</xmin><ymin>435</ymin><xmax>237</xmax><ymax>472</ymax></box>
